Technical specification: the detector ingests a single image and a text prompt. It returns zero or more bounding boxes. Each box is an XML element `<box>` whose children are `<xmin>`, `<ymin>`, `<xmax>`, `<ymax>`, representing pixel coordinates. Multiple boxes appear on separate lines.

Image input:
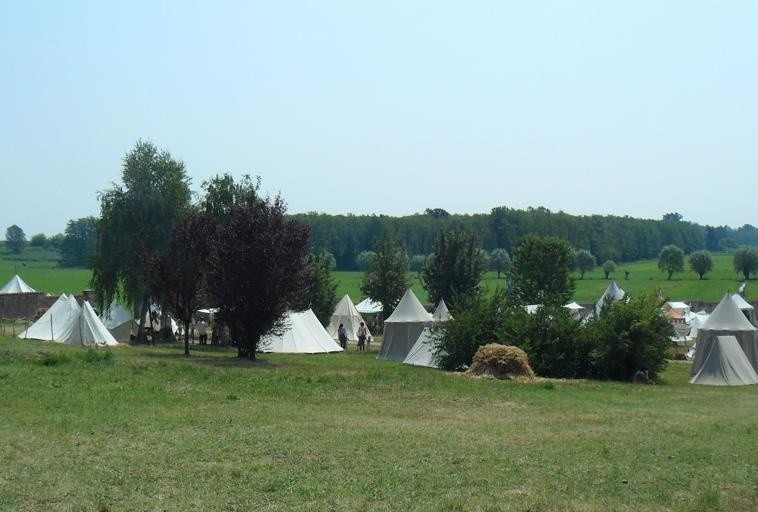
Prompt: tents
<box><xmin>0</xmin><ymin>275</ymin><xmax>36</xmax><ymax>293</ymax></box>
<box><xmin>497</xmin><ymin>278</ymin><xmax>758</xmax><ymax>386</ymax></box>
